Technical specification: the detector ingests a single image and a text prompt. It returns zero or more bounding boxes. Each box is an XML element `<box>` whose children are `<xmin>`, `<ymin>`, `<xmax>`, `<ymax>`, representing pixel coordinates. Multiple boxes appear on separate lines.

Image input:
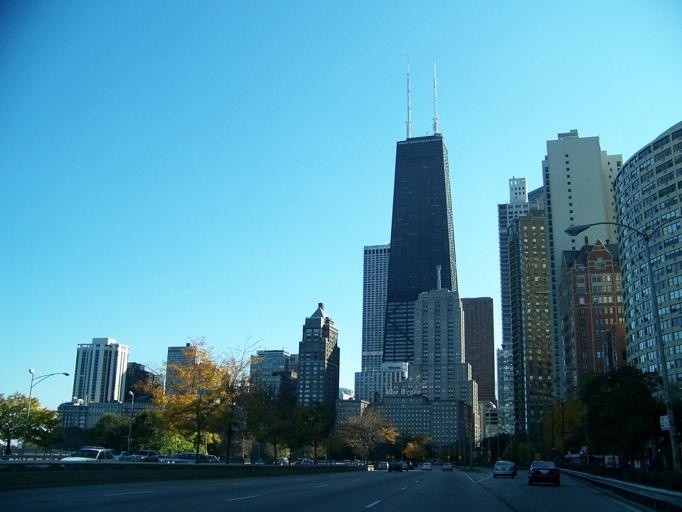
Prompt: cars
<box><xmin>339</xmin><ymin>457</ymin><xmax>434</xmax><ymax>472</ymax></box>
<box><xmin>441</xmin><ymin>462</ymin><xmax>455</xmax><ymax>472</ymax></box>
<box><xmin>55</xmin><ymin>444</ymin><xmax>325</xmax><ymax>465</ymax></box>
<box><xmin>492</xmin><ymin>460</ymin><xmax>517</xmax><ymax>479</ymax></box>
<box><xmin>0</xmin><ymin>439</ymin><xmax>23</xmax><ymax>458</ymax></box>
<box><xmin>526</xmin><ymin>460</ymin><xmax>562</xmax><ymax>487</ymax></box>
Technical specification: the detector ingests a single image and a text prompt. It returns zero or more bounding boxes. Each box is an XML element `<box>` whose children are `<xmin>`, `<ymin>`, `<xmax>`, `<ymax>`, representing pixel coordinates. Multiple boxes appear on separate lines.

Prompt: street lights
<box><xmin>127</xmin><ymin>390</ymin><xmax>136</xmax><ymax>453</ymax></box>
<box><xmin>21</xmin><ymin>367</ymin><xmax>71</xmax><ymax>457</ymax></box>
<box><xmin>563</xmin><ymin>221</ymin><xmax>681</xmax><ymax>473</ymax></box>
<box><xmin>516</xmin><ymin>365</ymin><xmax>599</xmax><ymax>456</ymax></box>
<box><xmin>226</xmin><ymin>399</ymin><xmax>237</xmax><ymax>465</ymax></box>
<box><xmin>487</xmin><ymin>403</ymin><xmax>494</xmax><ymax>464</ymax></box>
